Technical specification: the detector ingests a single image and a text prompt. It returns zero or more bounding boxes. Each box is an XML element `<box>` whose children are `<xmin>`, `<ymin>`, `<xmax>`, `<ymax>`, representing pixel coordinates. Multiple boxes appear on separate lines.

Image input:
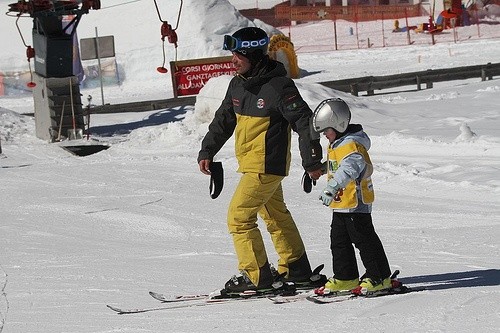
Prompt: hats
<box><xmin>232</xmin><ymin>27</ymin><xmax>268</xmax><ymax>59</ymax></box>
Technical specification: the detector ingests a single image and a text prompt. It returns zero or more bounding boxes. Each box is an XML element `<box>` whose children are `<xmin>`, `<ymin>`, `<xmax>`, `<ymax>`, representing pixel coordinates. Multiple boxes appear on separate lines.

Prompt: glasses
<box><xmin>223</xmin><ymin>34</ymin><xmax>268</xmax><ymax>51</ymax></box>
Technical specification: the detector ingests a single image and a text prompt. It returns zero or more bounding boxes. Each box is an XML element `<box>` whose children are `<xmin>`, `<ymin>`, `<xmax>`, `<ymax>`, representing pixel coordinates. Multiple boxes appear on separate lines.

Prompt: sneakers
<box><xmin>225</xmin><ymin>271</ymin><xmax>273</xmax><ymax>292</ymax></box>
<box><xmin>323</xmin><ymin>276</ymin><xmax>360</xmax><ymax>297</ymax></box>
<box><xmin>358</xmin><ymin>269</ymin><xmax>393</xmax><ymax>297</ymax></box>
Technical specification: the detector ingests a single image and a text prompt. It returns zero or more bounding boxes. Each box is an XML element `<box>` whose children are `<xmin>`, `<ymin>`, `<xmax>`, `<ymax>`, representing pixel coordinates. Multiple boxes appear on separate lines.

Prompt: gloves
<box><xmin>300</xmin><ymin>169</ymin><xmax>317</xmax><ymax>193</ymax></box>
<box><xmin>207</xmin><ymin>161</ymin><xmax>224</xmax><ymax>198</ymax></box>
<box><xmin>319</xmin><ymin>186</ymin><xmax>336</xmax><ymax>206</ymax></box>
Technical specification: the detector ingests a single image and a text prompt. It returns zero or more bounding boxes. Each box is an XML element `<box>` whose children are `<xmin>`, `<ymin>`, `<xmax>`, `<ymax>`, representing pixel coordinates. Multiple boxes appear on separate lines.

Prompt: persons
<box><xmin>197</xmin><ymin>27</ymin><xmax>323</xmax><ymax>299</ymax></box>
<box><xmin>307</xmin><ymin>98</ymin><xmax>402</xmax><ymax>303</ymax></box>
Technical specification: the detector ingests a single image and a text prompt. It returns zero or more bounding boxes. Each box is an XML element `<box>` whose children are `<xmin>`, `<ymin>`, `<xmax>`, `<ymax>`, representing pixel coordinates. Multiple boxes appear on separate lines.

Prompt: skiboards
<box><xmin>106</xmin><ymin>264</ymin><xmax>329</xmax><ymax>315</ymax></box>
<box><xmin>268</xmin><ymin>269</ymin><xmax>427</xmax><ymax>305</ymax></box>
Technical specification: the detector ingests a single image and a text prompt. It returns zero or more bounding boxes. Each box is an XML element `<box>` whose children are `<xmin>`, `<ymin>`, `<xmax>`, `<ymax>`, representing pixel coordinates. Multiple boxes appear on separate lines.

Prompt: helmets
<box><xmin>312</xmin><ymin>98</ymin><xmax>353</xmax><ymax>134</ymax></box>
